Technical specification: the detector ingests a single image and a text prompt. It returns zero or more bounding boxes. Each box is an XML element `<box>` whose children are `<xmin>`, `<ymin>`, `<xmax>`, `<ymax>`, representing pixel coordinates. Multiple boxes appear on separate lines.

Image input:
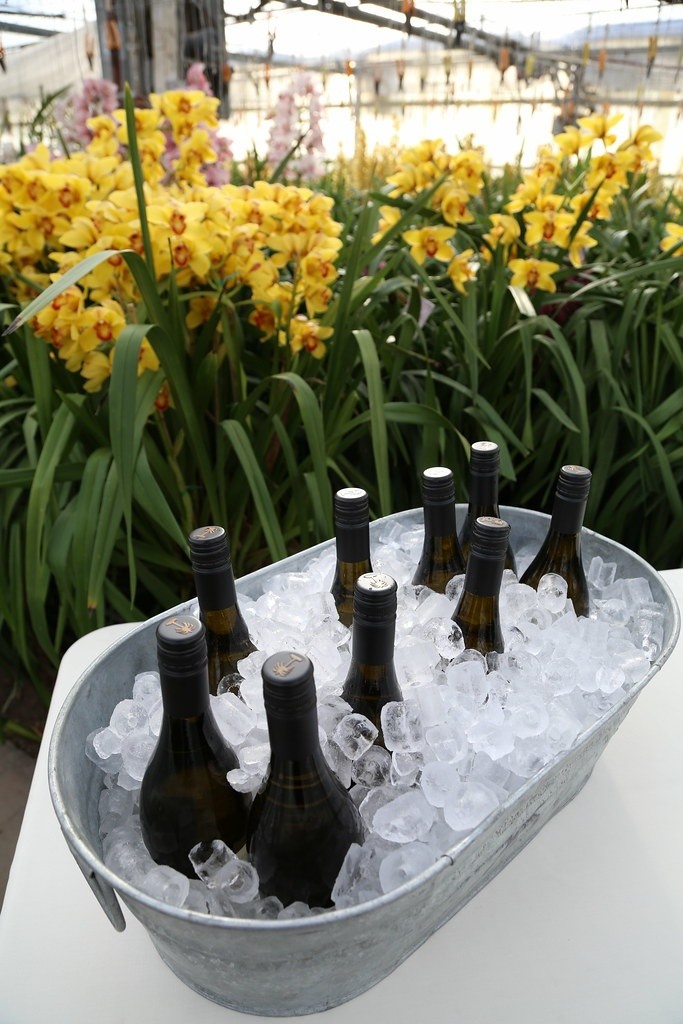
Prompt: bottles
<box><xmin>344</xmin><ymin>573</ymin><xmax>405</xmax><ymax>750</ymax></box>
<box><xmin>412</xmin><ymin>465</ymin><xmax>465</xmax><ymax>593</ymax></box>
<box><xmin>140</xmin><ymin>616</ymin><xmax>253</xmax><ymax>880</ymax></box>
<box><xmin>330</xmin><ymin>487</ymin><xmax>374</xmax><ymax>628</ymax></box>
<box><xmin>190</xmin><ymin>525</ymin><xmax>259</xmax><ymax>698</ymax></box>
<box><xmin>451</xmin><ymin>516</ymin><xmax>511</xmax><ymax>655</ymax></box>
<box><xmin>459</xmin><ymin>441</ymin><xmax>517</xmax><ymax>575</ymax></box>
<box><xmin>248</xmin><ymin>654</ymin><xmax>365</xmax><ymax>910</ymax></box>
<box><xmin>519</xmin><ymin>464</ymin><xmax>591</xmax><ymax>618</ymax></box>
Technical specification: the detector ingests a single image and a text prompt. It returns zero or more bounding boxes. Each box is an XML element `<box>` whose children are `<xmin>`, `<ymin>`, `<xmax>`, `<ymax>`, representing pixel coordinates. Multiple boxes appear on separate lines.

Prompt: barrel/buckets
<box><xmin>45</xmin><ymin>502</ymin><xmax>681</xmax><ymax>1015</ymax></box>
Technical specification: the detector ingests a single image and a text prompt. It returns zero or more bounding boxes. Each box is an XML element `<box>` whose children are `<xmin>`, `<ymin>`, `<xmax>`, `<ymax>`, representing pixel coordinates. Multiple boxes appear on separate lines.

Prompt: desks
<box><xmin>0</xmin><ymin>568</ymin><xmax>683</xmax><ymax>1023</ymax></box>
